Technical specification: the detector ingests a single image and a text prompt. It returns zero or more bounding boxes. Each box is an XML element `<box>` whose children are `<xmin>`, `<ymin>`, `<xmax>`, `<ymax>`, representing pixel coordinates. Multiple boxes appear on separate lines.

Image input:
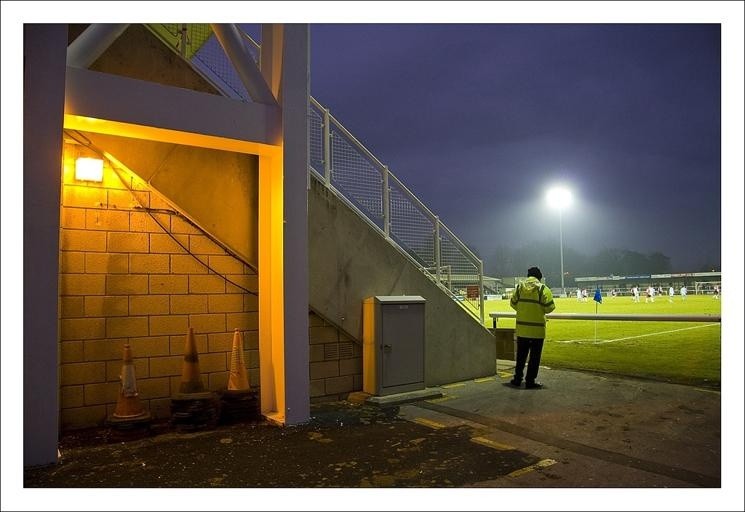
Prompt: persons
<box><xmin>576</xmin><ymin>283</ymin><xmax>721</xmax><ymax>304</ymax></box>
<box><xmin>510</xmin><ymin>265</ymin><xmax>555</xmax><ymax>389</ymax></box>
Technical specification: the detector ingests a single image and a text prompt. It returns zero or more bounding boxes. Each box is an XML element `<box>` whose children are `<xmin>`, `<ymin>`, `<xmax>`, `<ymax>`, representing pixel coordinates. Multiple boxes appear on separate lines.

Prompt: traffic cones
<box><xmin>168</xmin><ymin>327</ymin><xmax>220</xmax><ymax>431</ymax></box>
<box><xmin>104</xmin><ymin>344</ymin><xmax>154</xmax><ymax>442</ymax></box>
<box><xmin>216</xmin><ymin>327</ymin><xmax>260</xmax><ymax>425</ymax></box>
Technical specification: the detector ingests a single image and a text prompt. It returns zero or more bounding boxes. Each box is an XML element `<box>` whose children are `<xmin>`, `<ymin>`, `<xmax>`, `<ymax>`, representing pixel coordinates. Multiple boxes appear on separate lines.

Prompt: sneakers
<box><xmin>526</xmin><ymin>383</ymin><xmax>543</xmax><ymax>388</ymax></box>
<box><xmin>511</xmin><ymin>380</ymin><xmax>521</xmax><ymax>387</ymax></box>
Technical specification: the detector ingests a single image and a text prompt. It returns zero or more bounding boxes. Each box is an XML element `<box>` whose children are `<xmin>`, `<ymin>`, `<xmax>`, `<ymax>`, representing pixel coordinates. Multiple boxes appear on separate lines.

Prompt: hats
<box><xmin>528</xmin><ymin>267</ymin><xmax>542</xmax><ymax>278</ymax></box>
